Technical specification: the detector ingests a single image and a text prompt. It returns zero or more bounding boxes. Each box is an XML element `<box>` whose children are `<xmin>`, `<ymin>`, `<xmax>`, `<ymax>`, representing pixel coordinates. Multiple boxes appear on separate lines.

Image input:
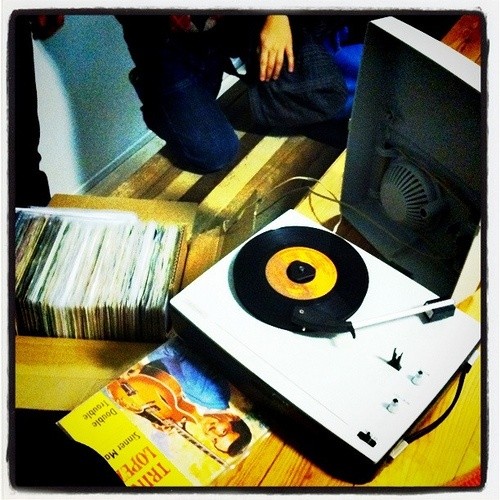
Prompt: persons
<box><xmin>115</xmin><ymin>12</ymin><xmax>350</xmax><ymax>172</ymax></box>
<box><xmin>12</xmin><ymin>13</ymin><xmax>68</xmax><ymax>210</ymax></box>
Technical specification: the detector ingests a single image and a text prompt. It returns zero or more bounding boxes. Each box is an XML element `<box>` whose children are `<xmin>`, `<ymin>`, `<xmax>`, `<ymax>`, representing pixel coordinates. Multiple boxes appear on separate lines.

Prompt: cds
<box><xmin>13</xmin><ymin>208</ymin><xmax>179</xmax><ymax>337</ymax></box>
<box><xmin>232</xmin><ymin>226</ymin><xmax>369</xmax><ymax>332</ymax></box>
